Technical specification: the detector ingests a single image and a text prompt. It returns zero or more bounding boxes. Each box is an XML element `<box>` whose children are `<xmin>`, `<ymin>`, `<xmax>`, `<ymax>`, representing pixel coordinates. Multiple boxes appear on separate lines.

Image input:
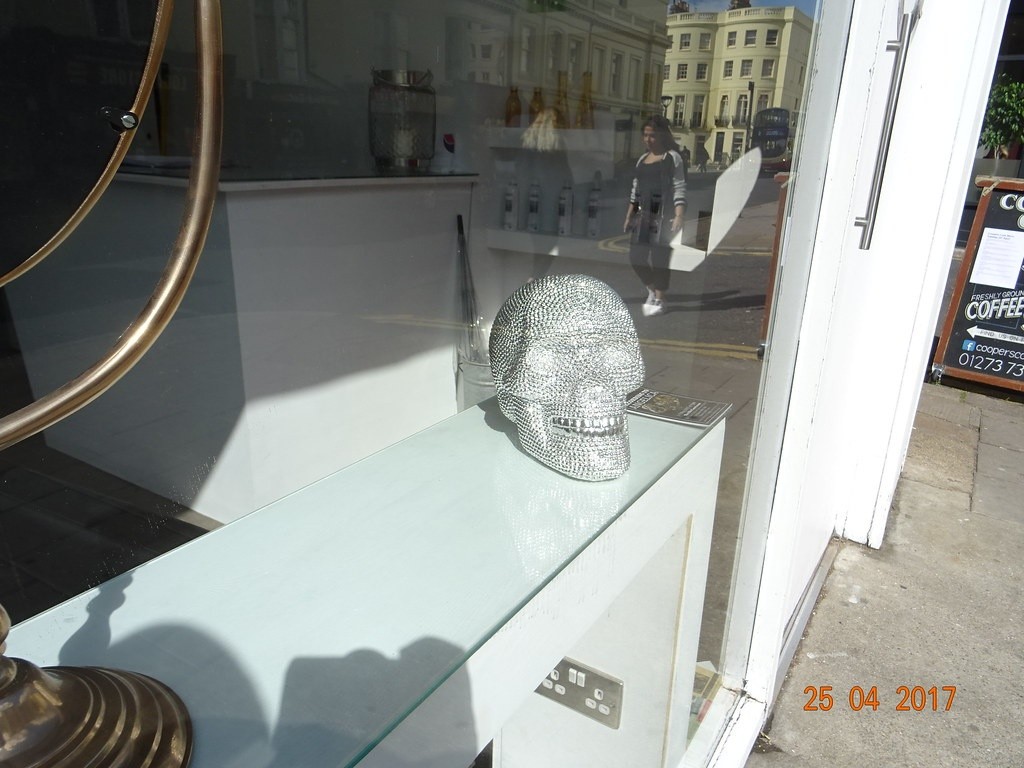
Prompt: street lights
<box><xmin>662</xmin><ymin>95</ymin><xmax>673</xmax><ymax>119</ymax></box>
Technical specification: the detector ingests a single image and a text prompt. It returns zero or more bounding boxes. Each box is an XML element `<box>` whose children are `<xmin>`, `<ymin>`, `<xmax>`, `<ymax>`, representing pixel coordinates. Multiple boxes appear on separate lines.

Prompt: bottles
<box><xmin>505</xmin><ymin>69</ymin><xmax>596</xmax><ymax>128</ymax></box>
<box><xmin>504</xmin><ymin>184</ymin><xmax>664</xmax><ymax>244</ymax></box>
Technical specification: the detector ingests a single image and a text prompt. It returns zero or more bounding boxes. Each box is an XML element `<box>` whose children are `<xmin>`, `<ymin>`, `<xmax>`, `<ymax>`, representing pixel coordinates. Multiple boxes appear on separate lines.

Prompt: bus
<box><xmin>752</xmin><ymin>107</ymin><xmax>800</xmax><ymax>174</ymax></box>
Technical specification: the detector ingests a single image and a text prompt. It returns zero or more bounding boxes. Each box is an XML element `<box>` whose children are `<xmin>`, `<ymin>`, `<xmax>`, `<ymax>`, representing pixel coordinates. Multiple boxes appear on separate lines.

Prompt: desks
<box><xmin>3</xmin><ymin>387</ymin><xmax>733</xmax><ymax>768</ymax></box>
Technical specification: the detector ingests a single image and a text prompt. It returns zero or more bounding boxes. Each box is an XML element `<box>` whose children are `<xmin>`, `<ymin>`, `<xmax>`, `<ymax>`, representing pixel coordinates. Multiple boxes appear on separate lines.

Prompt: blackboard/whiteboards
<box><xmin>932</xmin><ymin>173</ymin><xmax>1024</xmax><ymax>393</ymax></box>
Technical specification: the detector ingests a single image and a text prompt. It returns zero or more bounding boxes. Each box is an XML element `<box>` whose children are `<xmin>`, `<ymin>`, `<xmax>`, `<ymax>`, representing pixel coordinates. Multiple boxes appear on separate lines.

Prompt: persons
<box><xmin>682</xmin><ymin>145</ymin><xmax>710</xmax><ymax>173</ymax></box>
<box><xmin>513</xmin><ymin>107</ymin><xmax>575</xmax><ymax>277</ymax></box>
<box><xmin>624</xmin><ymin>114</ymin><xmax>687</xmax><ymax>315</ymax></box>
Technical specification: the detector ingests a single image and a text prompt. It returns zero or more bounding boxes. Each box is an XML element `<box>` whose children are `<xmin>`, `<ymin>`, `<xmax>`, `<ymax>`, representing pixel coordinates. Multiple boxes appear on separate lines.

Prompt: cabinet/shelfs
<box><xmin>0</xmin><ymin>159</ymin><xmax>481</xmax><ymax>524</ymax></box>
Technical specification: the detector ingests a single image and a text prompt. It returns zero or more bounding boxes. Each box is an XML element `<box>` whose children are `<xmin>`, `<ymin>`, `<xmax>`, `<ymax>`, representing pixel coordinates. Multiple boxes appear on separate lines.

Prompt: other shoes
<box><xmin>642</xmin><ymin>299</ymin><xmax>667</xmax><ymax>316</ymax></box>
